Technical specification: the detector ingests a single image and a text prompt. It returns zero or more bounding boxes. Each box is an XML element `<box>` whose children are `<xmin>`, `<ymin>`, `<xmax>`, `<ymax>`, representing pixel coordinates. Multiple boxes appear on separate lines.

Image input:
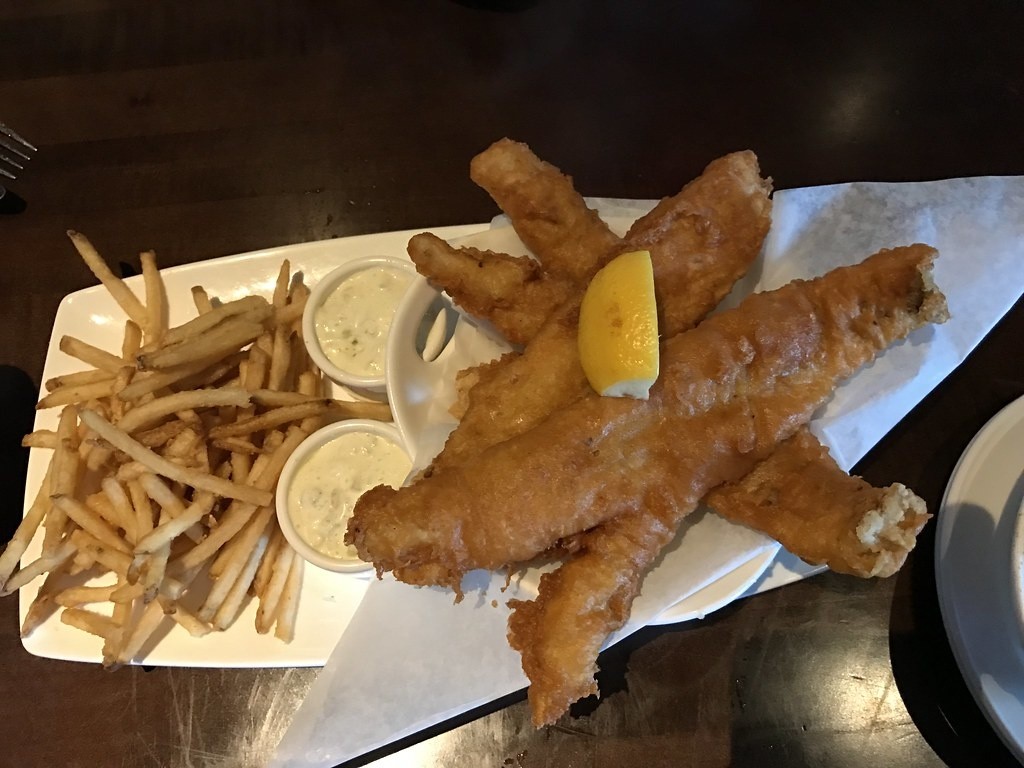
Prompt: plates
<box><xmin>18</xmin><ymin>222</ymin><xmax>489</xmax><ymax>672</ymax></box>
<box><xmin>386</xmin><ymin>231</ymin><xmax>780</xmax><ymax>622</ymax></box>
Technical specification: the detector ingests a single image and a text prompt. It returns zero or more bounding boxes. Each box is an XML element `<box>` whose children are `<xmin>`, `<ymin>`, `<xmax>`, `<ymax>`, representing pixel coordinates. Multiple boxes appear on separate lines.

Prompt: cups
<box><xmin>275</xmin><ymin>419</ymin><xmax>409</xmax><ymax>574</ymax></box>
<box><xmin>302</xmin><ymin>256</ymin><xmax>450</xmax><ymax>403</ymax></box>
<box><xmin>933</xmin><ymin>395</ymin><xmax>1024</xmax><ymax>767</ymax></box>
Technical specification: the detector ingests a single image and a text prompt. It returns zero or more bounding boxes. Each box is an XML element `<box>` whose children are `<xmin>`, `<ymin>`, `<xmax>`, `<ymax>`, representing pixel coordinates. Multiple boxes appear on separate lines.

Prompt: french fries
<box><xmin>0</xmin><ymin>230</ymin><xmax>393</xmax><ymax>671</ymax></box>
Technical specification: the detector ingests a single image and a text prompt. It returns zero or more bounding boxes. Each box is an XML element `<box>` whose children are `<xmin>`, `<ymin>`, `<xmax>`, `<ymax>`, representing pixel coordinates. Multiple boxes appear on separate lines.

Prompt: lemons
<box><xmin>578</xmin><ymin>251</ymin><xmax>660</xmax><ymax>400</ymax></box>
